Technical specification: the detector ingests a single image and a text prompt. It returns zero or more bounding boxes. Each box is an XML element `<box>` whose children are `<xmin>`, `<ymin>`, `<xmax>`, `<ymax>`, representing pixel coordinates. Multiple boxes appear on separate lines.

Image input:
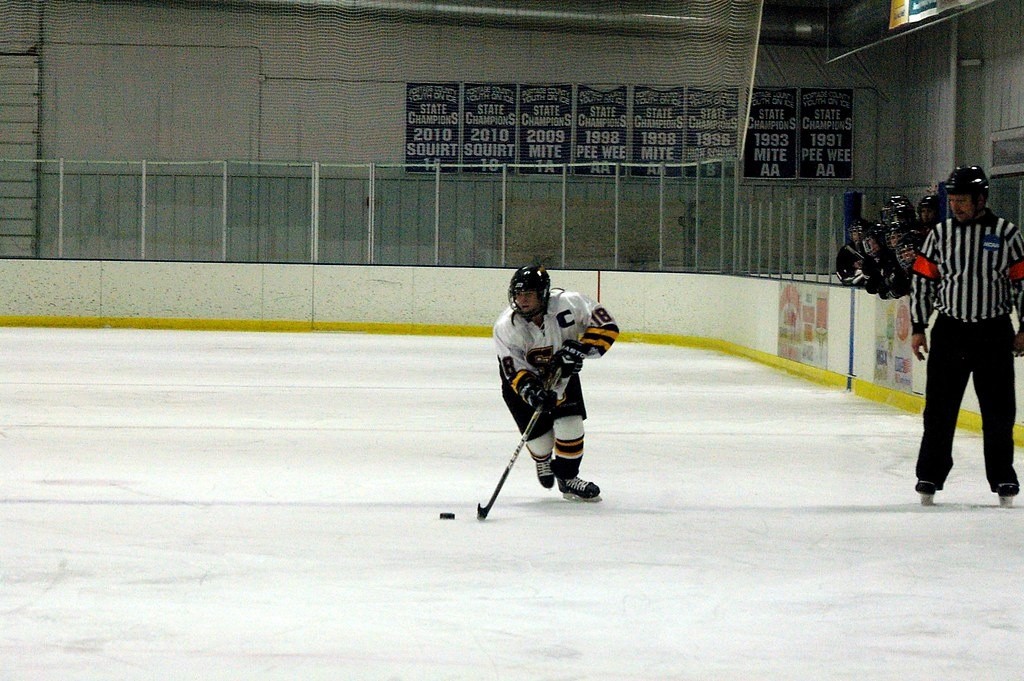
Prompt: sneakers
<box><xmin>995</xmin><ymin>481</ymin><xmax>1020</xmax><ymax>508</ymax></box>
<box><xmin>526</xmin><ymin>443</ymin><xmax>555</xmax><ymax>491</ymax></box>
<box><xmin>549</xmin><ymin>459</ymin><xmax>602</xmax><ymax>502</ymax></box>
<box><xmin>915</xmin><ymin>478</ymin><xmax>937</xmax><ymax>506</ymax></box>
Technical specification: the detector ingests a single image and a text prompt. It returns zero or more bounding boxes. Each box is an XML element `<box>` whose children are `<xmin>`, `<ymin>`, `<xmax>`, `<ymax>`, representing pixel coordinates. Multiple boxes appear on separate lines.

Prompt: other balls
<box><xmin>439</xmin><ymin>512</ymin><xmax>456</xmax><ymax>520</ymax></box>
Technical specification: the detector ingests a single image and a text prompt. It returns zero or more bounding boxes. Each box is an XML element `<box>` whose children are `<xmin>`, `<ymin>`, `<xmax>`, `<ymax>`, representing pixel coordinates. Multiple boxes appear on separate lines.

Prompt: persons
<box><xmin>910</xmin><ymin>164</ymin><xmax>1024</xmax><ymax>509</ymax></box>
<box><xmin>493</xmin><ymin>266</ymin><xmax>619</xmax><ymax>503</ymax></box>
<box><xmin>836</xmin><ymin>194</ymin><xmax>942</xmax><ymax>301</ymax></box>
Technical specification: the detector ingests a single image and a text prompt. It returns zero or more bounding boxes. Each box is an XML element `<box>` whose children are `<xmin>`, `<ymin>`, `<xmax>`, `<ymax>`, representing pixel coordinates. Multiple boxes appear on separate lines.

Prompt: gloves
<box><xmin>861</xmin><ymin>265</ymin><xmax>909</xmax><ymax>300</ymax></box>
<box><xmin>515</xmin><ymin>372</ymin><xmax>558</xmax><ymax>413</ymax></box>
<box><xmin>547</xmin><ymin>339</ymin><xmax>592</xmax><ymax>378</ymax></box>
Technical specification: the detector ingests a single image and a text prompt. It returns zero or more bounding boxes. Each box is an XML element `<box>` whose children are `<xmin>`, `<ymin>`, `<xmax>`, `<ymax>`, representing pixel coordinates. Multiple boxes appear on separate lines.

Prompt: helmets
<box><xmin>847</xmin><ymin>195</ymin><xmax>930</xmax><ymax>270</ymax></box>
<box><xmin>917</xmin><ymin>196</ymin><xmax>940</xmax><ymax>216</ymax></box>
<box><xmin>944</xmin><ymin>164</ymin><xmax>988</xmax><ymax>201</ymax></box>
<box><xmin>508</xmin><ymin>264</ymin><xmax>550</xmax><ymax>318</ymax></box>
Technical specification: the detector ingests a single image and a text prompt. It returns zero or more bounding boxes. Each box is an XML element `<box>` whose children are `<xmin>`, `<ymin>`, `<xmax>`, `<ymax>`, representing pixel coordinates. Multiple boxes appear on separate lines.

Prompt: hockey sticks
<box><xmin>476</xmin><ymin>364</ymin><xmax>565</xmax><ymax>521</ymax></box>
